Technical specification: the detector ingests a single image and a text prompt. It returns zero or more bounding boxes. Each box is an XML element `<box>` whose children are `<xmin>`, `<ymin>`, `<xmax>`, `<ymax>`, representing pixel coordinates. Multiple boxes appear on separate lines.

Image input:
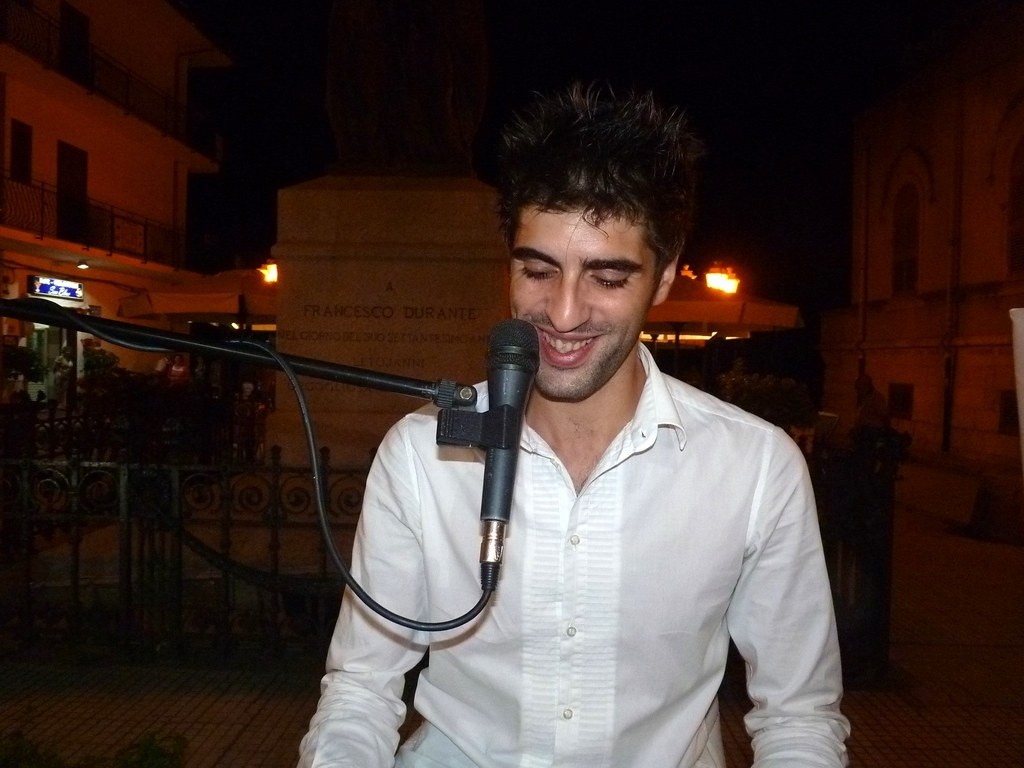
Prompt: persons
<box><xmin>167</xmin><ymin>353</ymin><xmax>188</xmax><ymax>389</ymax></box>
<box><xmin>45</xmin><ymin>356</ymin><xmax>67</xmax><ymax>426</ymax></box>
<box><xmin>710</xmin><ymin>358</ymin><xmax>780</xmax><ymax>410</ymax></box>
<box><xmin>852</xmin><ymin>375</ymin><xmax>892</xmax><ymax>438</ymax></box>
<box><xmin>296</xmin><ymin>71</ymin><xmax>847</xmax><ymax>768</ymax></box>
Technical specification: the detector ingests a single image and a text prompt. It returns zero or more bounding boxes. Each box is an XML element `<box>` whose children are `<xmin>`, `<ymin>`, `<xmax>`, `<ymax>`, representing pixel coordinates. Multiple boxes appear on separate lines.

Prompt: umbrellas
<box><xmin>119</xmin><ymin>266</ymin><xmax>280</xmax><ymax>343</ymax></box>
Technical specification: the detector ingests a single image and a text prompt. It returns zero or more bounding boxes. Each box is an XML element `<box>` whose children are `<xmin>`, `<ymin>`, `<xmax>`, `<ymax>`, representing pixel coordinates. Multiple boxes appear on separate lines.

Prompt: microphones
<box><xmin>479</xmin><ymin>317</ymin><xmax>541</xmax><ymax>590</ymax></box>
